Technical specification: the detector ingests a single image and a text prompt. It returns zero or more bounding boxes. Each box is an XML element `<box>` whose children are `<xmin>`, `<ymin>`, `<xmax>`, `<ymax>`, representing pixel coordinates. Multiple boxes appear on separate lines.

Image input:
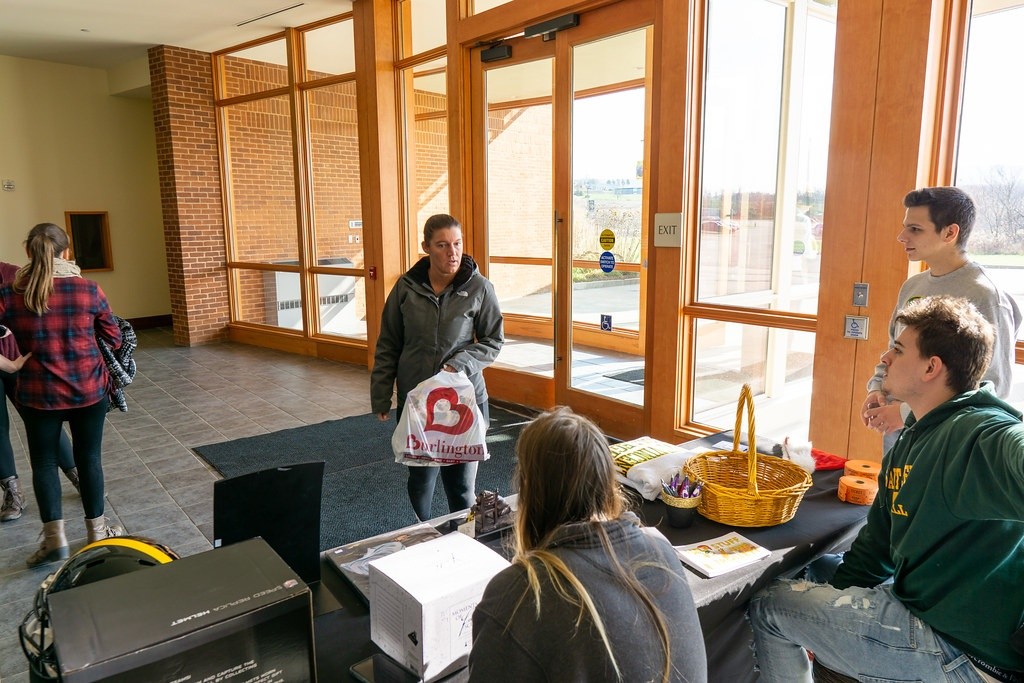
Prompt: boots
<box><xmin>25</xmin><ymin>519</ymin><xmax>70</xmax><ymax>566</ymax></box>
<box><xmin>0</xmin><ymin>475</ymin><xmax>27</xmax><ymax>521</ymax></box>
<box><xmin>84</xmin><ymin>513</ymin><xmax>122</xmax><ymax>545</ymax></box>
<box><xmin>65</xmin><ymin>468</ymin><xmax>108</xmax><ymax>498</ymax></box>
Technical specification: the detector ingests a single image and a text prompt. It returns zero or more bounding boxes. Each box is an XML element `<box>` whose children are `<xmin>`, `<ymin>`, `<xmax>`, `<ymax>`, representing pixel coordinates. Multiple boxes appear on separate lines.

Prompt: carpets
<box><xmin>191</xmin><ymin>395</ymin><xmax>622</xmax><ymax>552</ymax></box>
<box><xmin>602</xmin><ymin>358</ymin><xmax>750</xmax><ymax>398</ymax></box>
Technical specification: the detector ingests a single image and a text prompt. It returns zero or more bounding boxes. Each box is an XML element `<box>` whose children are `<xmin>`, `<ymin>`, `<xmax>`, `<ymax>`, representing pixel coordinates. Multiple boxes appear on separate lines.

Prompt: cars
<box><xmin>701</xmin><ymin>216</ymin><xmax>741</xmax><ymax>233</ymax></box>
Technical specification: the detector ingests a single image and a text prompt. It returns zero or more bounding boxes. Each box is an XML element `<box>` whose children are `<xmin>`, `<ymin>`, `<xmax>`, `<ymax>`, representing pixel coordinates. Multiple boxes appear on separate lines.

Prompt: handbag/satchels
<box><xmin>391</xmin><ymin>366</ymin><xmax>491</xmax><ymax>467</ymax></box>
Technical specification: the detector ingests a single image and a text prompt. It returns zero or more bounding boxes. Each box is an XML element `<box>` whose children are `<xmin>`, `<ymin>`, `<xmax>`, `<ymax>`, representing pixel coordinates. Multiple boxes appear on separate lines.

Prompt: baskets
<box><xmin>683</xmin><ymin>384</ymin><xmax>813</xmax><ymax>528</ymax></box>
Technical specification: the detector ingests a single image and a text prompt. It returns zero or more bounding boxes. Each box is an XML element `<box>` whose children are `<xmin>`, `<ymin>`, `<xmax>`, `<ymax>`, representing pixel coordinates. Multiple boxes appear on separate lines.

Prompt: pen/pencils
<box><xmin>659</xmin><ymin>470</ymin><xmax>704</xmax><ymax>498</ymax></box>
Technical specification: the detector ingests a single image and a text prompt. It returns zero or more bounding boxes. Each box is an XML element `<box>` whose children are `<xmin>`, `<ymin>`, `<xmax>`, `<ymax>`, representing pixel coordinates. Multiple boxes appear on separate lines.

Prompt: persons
<box><xmin>0</xmin><ymin>258</ymin><xmax>108</xmax><ymax>522</ymax></box>
<box><xmin>745</xmin><ymin>296</ymin><xmax>1024</xmax><ymax>683</ymax></box>
<box><xmin>369</xmin><ymin>212</ymin><xmax>506</xmax><ymax>531</ymax></box>
<box><xmin>464</xmin><ymin>405</ymin><xmax>708</xmax><ymax>683</ymax></box>
<box><xmin>0</xmin><ymin>222</ymin><xmax>123</xmax><ymax>570</ymax></box>
<box><xmin>860</xmin><ymin>185</ymin><xmax>1023</xmax><ymax>466</ymax></box>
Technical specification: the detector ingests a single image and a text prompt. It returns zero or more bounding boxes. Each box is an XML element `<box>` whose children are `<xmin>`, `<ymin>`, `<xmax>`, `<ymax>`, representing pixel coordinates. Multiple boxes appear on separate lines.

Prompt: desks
<box><xmin>308</xmin><ymin>429</ymin><xmax>880</xmax><ymax>683</ymax></box>
